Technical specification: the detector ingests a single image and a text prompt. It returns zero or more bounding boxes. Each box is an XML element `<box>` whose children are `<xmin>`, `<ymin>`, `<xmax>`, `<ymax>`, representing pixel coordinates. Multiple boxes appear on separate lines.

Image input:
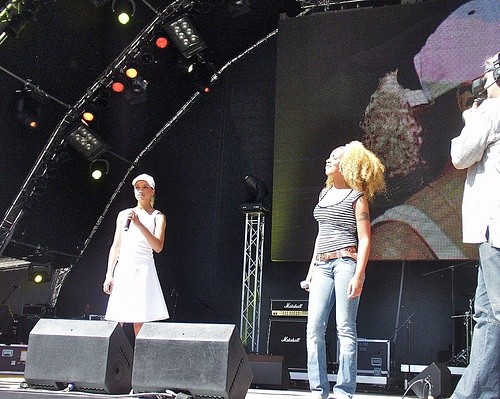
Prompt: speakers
<box><xmin>25</xmin><ymin>317</ymin><xmax>135</xmax><ymax>395</ymax></box>
<box><xmin>408</xmin><ymin>363</ymin><xmax>451</xmax><ymax>398</ymax></box>
<box><xmin>247</xmin><ymin>355</ymin><xmax>291</xmax><ymax>391</ymax></box>
<box><xmin>131</xmin><ymin>322</ymin><xmax>254</xmax><ymax>399</ymax></box>
<box><xmin>266</xmin><ymin>317</ymin><xmax>308</xmax><ymax>370</ymax></box>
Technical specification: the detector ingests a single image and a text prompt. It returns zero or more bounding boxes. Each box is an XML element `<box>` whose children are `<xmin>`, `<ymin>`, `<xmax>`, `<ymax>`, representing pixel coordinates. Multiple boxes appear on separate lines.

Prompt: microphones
<box><xmin>124</xmin><ymin>213</ymin><xmax>133</xmax><ymax>231</ymax></box>
<box><xmin>11</xmin><ymin>285</ymin><xmax>21</xmax><ymax>288</ymax></box>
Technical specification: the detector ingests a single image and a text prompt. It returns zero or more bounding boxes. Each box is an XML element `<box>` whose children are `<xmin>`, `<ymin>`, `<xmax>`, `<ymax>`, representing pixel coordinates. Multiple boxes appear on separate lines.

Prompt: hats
<box><xmin>132</xmin><ymin>174</ymin><xmax>155</xmax><ymax>190</ymax></box>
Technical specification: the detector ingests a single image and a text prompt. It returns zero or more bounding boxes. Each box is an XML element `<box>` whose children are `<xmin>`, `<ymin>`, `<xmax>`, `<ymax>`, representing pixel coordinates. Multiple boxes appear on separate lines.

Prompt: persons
<box><xmin>447</xmin><ymin>52</ymin><xmax>500</xmax><ymax>399</ymax></box>
<box><xmin>102</xmin><ymin>174</ymin><xmax>170</xmax><ymax>336</ymax></box>
<box><xmin>358</xmin><ymin>71</ymin><xmax>482</xmax><ymax>259</ymax></box>
<box><xmin>300</xmin><ymin>140</ymin><xmax>387</xmax><ymax>399</ymax></box>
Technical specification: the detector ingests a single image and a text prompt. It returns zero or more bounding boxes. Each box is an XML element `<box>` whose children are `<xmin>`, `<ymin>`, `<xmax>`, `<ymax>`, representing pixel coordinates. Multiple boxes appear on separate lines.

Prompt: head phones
<box><xmin>492</xmin><ymin>53</ymin><xmax>500</xmax><ymax>85</ymax></box>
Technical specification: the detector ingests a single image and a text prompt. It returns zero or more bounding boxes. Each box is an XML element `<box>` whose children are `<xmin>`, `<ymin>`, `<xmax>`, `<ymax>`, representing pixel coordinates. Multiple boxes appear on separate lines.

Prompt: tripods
<box><xmin>445</xmin><ymin>299</ymin><xmax>474</xmax><ymax>367</ymax></box>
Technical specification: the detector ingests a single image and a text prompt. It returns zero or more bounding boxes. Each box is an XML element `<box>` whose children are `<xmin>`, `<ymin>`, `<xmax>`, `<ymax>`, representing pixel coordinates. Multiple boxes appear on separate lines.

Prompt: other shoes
<box><xmin>327</xmin><ymin>392</ymin><xmax>349</xmax><ymax>399</ymax></box>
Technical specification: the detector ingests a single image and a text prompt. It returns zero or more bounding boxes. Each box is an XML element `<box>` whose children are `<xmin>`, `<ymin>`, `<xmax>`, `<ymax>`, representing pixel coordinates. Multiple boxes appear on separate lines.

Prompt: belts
<box><xmin>316</xmin><ymin>247</ymin><xmax>358</xmax><ymax>262</ymax></box>
